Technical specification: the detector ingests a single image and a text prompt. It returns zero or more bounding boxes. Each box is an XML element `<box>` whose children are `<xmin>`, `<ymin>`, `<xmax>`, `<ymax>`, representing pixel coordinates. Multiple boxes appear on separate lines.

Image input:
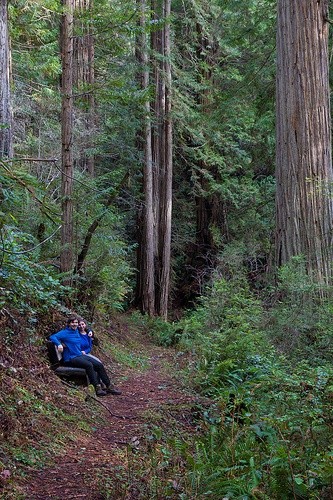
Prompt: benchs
<box><xmin>46</xmin><ymin>339</ymin><xmax>102</xmax><ymax>388</ymax></box>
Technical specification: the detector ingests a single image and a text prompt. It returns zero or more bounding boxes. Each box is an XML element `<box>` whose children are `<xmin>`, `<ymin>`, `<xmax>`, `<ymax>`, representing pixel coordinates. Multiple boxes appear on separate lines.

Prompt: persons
<box><xmin>49</xmin><ymin>317</ymin><xmax>123</xmax><ymax>396</ymax></box>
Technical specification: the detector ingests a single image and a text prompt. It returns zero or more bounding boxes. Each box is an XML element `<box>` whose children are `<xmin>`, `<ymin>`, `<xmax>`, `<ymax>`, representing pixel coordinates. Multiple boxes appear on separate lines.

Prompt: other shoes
<box><xmin>96</xmin><ymin>383</ymin><xmax>121</xmax><ymax>396</ymax></box>
